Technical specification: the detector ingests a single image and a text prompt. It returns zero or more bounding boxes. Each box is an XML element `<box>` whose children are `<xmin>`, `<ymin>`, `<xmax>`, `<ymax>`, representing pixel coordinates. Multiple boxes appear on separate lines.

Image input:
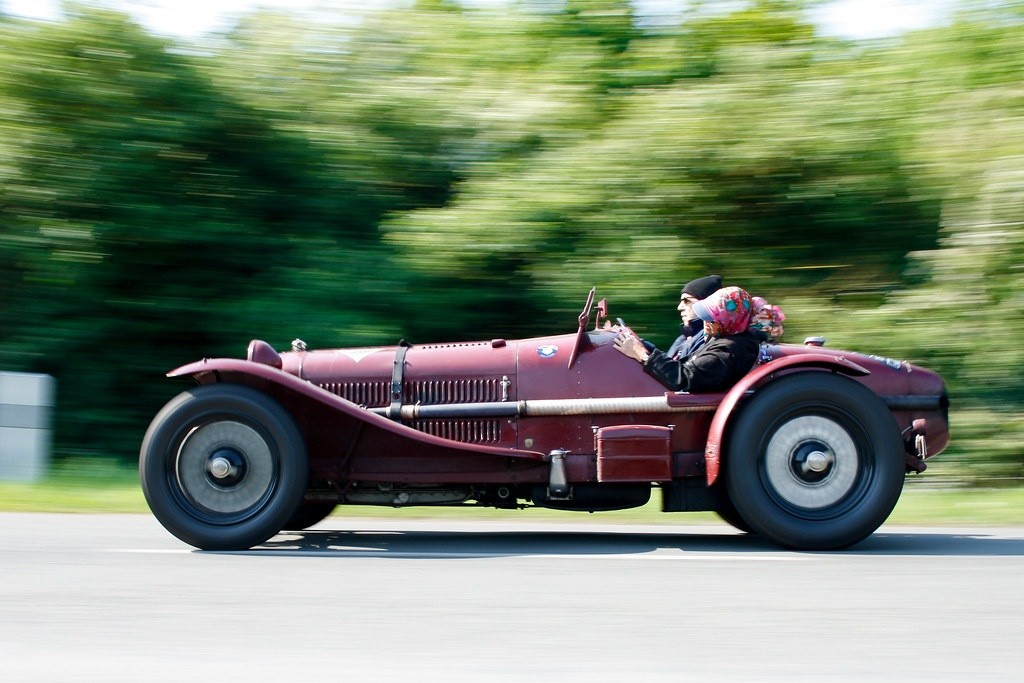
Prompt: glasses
<box><xmin>679</xmin><ymin>297</ymin><xmax>696</xmax><ymax>304</ymax></box>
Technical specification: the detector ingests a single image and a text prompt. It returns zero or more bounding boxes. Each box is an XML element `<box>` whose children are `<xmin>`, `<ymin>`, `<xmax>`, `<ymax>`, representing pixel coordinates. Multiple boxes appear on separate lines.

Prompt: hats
<box><xmin>691</xmin><ymin>302</ymin><xmax>714</xmax><ymax>322</ymax></box>
<box><xmin>681</xmin><ymin>274</ymin><xmax>723</xmax><ymax>301</ymax></box>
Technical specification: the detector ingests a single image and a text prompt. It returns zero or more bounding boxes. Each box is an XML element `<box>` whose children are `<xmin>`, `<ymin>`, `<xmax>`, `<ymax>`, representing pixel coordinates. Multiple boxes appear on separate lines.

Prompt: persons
<box><xmin>613</xmin><ymin>275</ymin><xmax>762</xmax><ymax>398</ymax></box>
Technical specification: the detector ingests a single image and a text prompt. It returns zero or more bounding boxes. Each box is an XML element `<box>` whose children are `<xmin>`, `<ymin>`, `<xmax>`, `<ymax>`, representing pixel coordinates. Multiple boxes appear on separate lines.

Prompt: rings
<box><xmin>620</xmin><ymin>338</ymin><xmax>625</xmax><ymax>342</ymax></box>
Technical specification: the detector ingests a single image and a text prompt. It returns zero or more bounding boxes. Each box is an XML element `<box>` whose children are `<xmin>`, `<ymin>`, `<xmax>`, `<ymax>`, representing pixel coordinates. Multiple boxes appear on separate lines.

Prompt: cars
<box><xmin>137</xmin><ymin>287</ymin><xmax>949</xmax><ymax>552</ymax></box>
